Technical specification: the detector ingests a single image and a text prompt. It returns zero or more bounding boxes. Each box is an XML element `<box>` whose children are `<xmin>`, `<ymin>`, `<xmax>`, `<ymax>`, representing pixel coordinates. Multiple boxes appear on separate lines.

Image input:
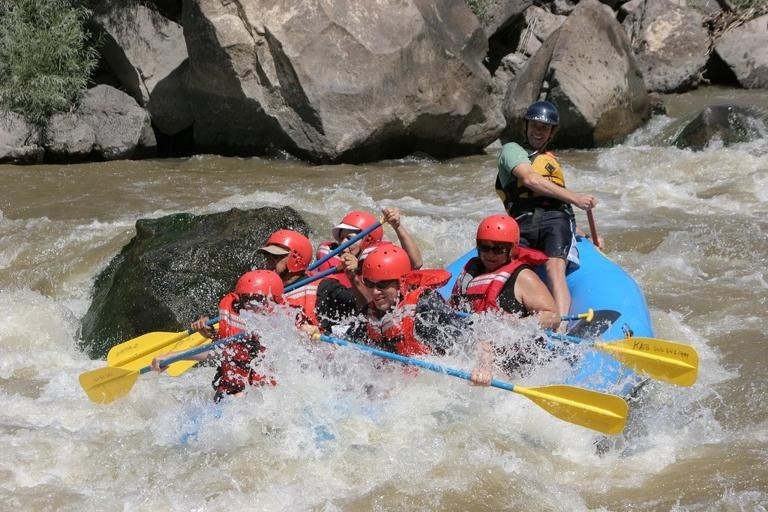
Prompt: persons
<box><xmin>493</xmin><ymin>101</ymin><xmax>606</xmax><ymax>335</ymax></box>
<box><xmin>153</xmin><ymin>208</ymin><xmax>493</xmax><ymax>392</ymax></box>
<box><xmin>446</xmin><ymin>213</ymin><xmax>560</xmax><ymax>335</ymax></box>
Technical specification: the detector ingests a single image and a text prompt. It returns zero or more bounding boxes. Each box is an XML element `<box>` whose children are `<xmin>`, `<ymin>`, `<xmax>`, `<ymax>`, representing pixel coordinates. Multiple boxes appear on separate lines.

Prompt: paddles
<box><xmin>79</xmin><ymin>332</ymin><xmax>253</xmax><ymax>406</ymax></box>
<box><xmin>107</xmin><ymin>263</ymin><xmax>346</xmax><ymax>370</ymax></box>
<box><xmin>455</xmin><ymin>309</ymin><xmax>698</xmax><ymax>387</ymax></box>
<box><xmin>309</xmin><ymin>331</ymin><xmax>628</xmax><ymax>435</ymax></box>
<box><xmin>165</xmin><ymin>219</ymin><xmax>387</xmax><ymax>376</ymax></box>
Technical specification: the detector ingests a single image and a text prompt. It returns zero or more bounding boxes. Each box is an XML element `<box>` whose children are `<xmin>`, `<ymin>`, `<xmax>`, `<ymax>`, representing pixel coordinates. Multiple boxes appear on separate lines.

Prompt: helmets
<box><xmin>363</xmin><ymin>244</ymin><xmax>411</xmax><ymax>281</ymax></box>
<box><xmin>234</xmin><ymin>269</ymin><xmax>285</xmax><ymax>303</ymax></box>
<box><xmin>477</xmin><ymin>214</ymin><xmax>520</xmax><ymax>249</ymax></box>
<box><xmin>525</xmin><ymin>101</ymin><xmax>560</xmax><ymax>126</ymax></box>
<box><xmin>341</xmin><ymin>211</ymin><xmax>384</xmax><ymax>248</ymax></box>
<box><xmin>267</xmin><ymin>229</ymin><xmax>313</xmax><ymax>273</ymax></box>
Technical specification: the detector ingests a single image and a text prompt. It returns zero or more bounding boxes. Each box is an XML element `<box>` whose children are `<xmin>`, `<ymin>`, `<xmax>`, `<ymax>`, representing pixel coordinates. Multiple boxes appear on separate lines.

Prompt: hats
<box><xmin>251</xmin><ymin>243</ymin><xmax>288</xmax><ymax>260</ymax></box>
<box><xmin>332</xmin><ymin>222</ymin><xmax>360</xmax><ymax>242</ymax></box>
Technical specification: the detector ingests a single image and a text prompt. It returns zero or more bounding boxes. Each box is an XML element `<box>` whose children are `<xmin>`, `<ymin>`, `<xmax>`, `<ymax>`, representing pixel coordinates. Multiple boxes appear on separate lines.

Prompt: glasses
<box><xmin>477</xmin><ymin>244</ymin><xmax>510</xmax><ymax>256</ymax></box>
<box><xmin>239</xmin><ymin>294</ymin><xmax>261</xmax><ymax>306</ymax></box>
<box><xmin>364</xmin><ymin>279</ymin><xmax>393</xmax><ymax>289</ymax></box>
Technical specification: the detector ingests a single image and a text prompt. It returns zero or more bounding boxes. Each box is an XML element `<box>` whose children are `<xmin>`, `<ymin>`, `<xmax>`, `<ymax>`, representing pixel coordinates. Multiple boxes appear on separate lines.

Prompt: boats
<box><xmin>89</xmin><ymin>228</ymin><xmax>654</xmax><ymax>478</ymax></box>
